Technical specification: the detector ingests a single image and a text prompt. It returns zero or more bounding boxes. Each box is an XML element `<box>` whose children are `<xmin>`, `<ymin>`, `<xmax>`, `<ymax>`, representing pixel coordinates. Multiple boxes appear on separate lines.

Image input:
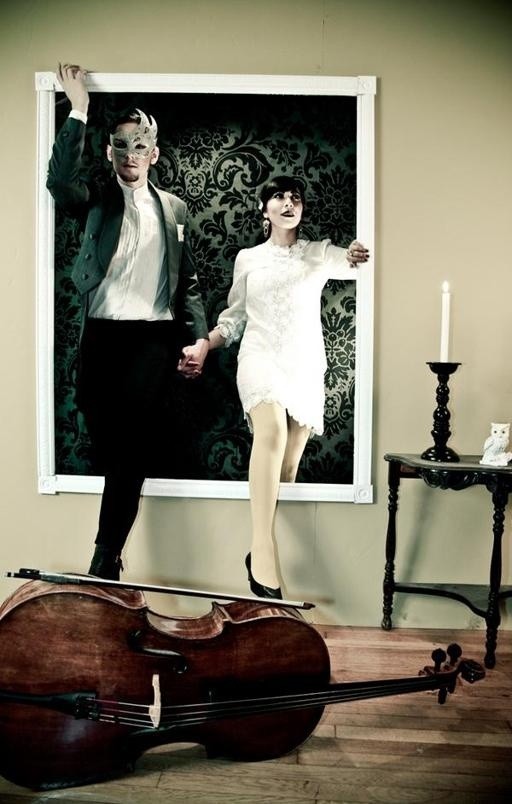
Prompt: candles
<box><xmin>438</xmin><ymin>281</ymin><xmax>451</xmax><ymax>360</ymax></box>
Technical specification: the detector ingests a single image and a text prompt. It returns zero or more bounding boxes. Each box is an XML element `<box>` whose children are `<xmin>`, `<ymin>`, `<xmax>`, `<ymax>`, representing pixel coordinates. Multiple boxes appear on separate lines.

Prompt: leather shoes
<box><xmin>87</xmin><ymin>547</ymin><xmax>124</xmax><ymax>581</ymax></box>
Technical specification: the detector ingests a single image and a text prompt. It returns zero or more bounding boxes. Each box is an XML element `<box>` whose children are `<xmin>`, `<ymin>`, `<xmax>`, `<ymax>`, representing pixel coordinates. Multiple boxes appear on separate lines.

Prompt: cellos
<box><xmin>1</xmin><ymin>564</ymin><xmax>484</xmax><ymax>792</ymax></box>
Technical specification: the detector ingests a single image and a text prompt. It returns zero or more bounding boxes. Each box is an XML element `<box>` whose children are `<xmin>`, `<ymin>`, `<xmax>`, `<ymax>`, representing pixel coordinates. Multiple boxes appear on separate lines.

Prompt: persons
<box><xmin>45</xmin><ymin>61</ymin><xmax>210</xmax><ymax>580</ymax></box>
<box><xmin>177</xmin><ymin>174</ymin><xmax>370</xmax><ymax>600</ymax></box>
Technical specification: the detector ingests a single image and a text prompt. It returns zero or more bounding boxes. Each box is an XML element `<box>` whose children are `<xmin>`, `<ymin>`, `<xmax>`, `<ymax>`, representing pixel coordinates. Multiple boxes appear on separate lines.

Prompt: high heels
<box><xmin>245</xmin><ymin>551</ymin><xmax>282</xmax><ymax>601</ymax></box>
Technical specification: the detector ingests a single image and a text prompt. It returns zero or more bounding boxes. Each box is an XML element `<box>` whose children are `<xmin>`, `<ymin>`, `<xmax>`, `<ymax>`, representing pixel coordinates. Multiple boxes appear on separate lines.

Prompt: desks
<box><xmin>378</xmin><ymin>455</ymin><xmax>512</xmax><ymax>670</ymax></box>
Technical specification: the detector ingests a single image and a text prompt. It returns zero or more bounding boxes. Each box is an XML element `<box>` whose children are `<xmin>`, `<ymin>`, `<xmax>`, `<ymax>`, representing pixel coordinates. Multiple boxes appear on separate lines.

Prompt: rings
<box><xmin>349</xmin><ymin>250</ymin><xmax>354</xmax><ymax>258</ymax></box>
